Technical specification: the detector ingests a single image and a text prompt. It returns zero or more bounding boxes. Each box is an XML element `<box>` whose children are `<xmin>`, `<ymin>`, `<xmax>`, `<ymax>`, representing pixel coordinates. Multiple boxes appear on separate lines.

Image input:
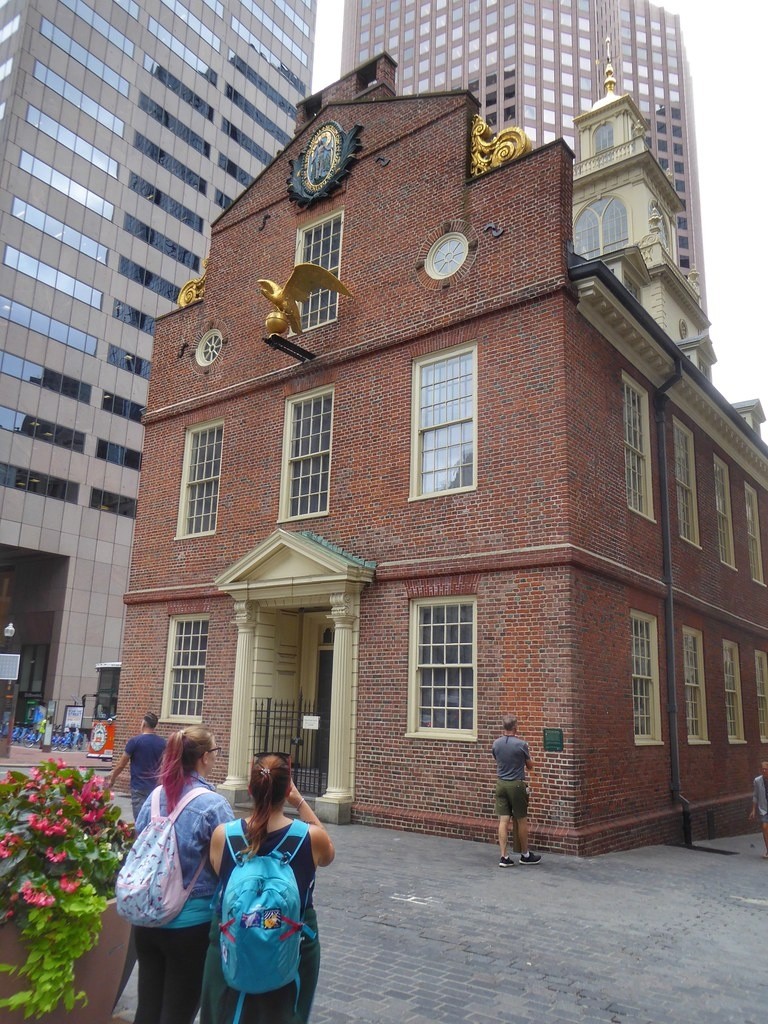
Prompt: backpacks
<box><xmin>116</xmin><ymin>784</ymin><xmax>218</xmax><ymax>926</ymax></box>
<box><xmin>218</xmin><ymin>818</ymin><xmax>317</xmax><ymax>994</ymax></box>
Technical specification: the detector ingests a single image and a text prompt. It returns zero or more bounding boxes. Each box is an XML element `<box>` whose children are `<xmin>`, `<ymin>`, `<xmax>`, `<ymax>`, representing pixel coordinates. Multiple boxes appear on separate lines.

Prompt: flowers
<box><xmin>0</xmin><ymin>760</ymin><xmax>141</xmax><ymax>1014</ymax></box>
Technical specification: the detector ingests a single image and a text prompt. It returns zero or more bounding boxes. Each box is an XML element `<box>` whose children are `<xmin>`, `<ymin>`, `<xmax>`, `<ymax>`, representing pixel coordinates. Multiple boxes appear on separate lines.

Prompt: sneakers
<box><xmin>499</xmin><ymin>856</ymin><xmax>514</xmax><ymax>867</ymax></box>
<box><xmin>519</xmin><ymin>852</ymin><xmax>541</xmax><ymax>865</ymax></box>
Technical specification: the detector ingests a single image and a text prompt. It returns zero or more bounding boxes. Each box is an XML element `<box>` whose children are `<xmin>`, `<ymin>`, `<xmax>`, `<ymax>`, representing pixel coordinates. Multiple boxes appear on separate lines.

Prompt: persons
<box><xmin>199</xmin><ymin>751</ymin><xmax>335</xmax><ymax>1024</ymax></box>
<box><xmin>748</xmin><ymin>759</ymin><xmax>768</xmax><ymax>858</ymax></box>
<box><xmin>39</xmin><ymin>718</ymin><xmax>46</xmax><ymax>749</ymax></box>
<box><xmin>107</xmin><ymin>713</ymin><xmax>168</xmax><ymax>822</ymax></box>
<box><xmin>134</xmin><ymin>725</ymin><xmax>236</xmax><ymax>1024</ymax></box>
<box><xmin>492</xmin><ymin>716</ymin><xmax>542</xmax><ymax>866</ymax></box>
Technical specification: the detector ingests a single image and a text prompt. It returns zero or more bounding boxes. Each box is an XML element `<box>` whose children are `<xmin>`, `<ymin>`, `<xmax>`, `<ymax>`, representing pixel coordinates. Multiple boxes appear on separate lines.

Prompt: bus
<box><xmin>81</xmin><ymin>662</ymin><xmax>122</xmax><ymax>762</ymax></box>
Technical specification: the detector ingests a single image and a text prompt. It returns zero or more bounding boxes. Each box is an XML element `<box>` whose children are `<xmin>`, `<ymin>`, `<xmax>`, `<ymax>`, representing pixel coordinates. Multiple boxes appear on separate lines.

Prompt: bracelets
<box><xmin>296</xmin><ymin>798</ymin><xmax>304</xmax><ymax>811</ymax></box>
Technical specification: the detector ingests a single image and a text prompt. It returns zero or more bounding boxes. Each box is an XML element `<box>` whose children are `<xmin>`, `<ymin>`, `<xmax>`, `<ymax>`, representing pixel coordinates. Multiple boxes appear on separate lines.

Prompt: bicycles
<box><xmin>0</xmin><ymin>721</ymin><xmax>83</xmax><ymax>752</ymax></box>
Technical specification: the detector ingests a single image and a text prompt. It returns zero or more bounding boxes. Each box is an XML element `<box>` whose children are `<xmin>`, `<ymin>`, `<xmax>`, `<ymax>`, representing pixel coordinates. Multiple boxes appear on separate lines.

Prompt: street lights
<box><xmin>3</xmin><ymin>621</ymin><xmax>16</xmax><ymax>653</ymax></box>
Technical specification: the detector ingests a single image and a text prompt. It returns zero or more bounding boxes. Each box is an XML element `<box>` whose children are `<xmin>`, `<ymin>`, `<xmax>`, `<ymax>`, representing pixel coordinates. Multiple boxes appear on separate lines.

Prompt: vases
<box><xmin>0</xmin><ymin>894</ymin><xmax>136</xmax><ymax>1024</ymax></box>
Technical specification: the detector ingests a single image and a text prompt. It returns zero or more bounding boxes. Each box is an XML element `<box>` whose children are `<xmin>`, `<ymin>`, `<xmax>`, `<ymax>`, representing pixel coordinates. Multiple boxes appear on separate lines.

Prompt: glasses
<box><xmin>251</xmin><ymin>752</ymin><xmax>291</xmax><ymax>787</ymax></box>
<box><xmin>199</xmin><ymin>747</ymin><xmax>222</xmax><ymax>759</ymax></box>
